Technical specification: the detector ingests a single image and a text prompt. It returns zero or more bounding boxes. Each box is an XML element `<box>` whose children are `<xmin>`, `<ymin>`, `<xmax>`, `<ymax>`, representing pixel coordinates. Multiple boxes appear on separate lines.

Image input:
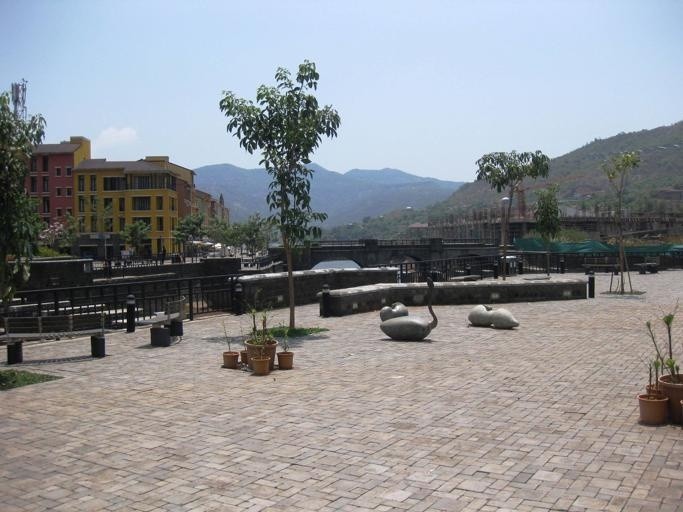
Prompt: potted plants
<box><xmin>635</xmin><ymin>295</ymin><xmax>683</xmax><ymax>426</ymax></box>
<box><xmin>221</xmin><ymin>289</ymin><xmax>294</xmax><ymax>377</ymax></box>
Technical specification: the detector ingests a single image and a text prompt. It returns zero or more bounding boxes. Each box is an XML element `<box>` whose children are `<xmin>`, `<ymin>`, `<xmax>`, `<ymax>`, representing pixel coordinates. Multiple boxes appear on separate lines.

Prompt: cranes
<box><xmin>516</xmin><ymin>175</ymin><xmax>577</xmax><ymax>216</ymax></box>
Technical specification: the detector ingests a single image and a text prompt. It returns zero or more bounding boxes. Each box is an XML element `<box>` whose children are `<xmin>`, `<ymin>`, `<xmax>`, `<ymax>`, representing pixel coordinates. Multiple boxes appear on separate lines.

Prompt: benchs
<box><xmin>0</xmin><ymin>312</ymin><xmax>113</xmax><ymax>364</ymax></box>
<box><xmin>137</xmin><ymin>295</ymin><xmax>185</xmax><ymax>347</ymax></box>
<box><xmin>582</xmin><ymin>255</ymin><xmax>661</xmax><ymax>277</ymax></box>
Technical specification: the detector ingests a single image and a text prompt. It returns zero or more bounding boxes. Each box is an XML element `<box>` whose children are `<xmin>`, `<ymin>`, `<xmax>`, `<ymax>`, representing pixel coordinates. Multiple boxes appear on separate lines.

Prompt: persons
<box><xmin>182</xmin><ymin>253</ymin><xmax>185</xmax><ymax>262</ymax></box>
